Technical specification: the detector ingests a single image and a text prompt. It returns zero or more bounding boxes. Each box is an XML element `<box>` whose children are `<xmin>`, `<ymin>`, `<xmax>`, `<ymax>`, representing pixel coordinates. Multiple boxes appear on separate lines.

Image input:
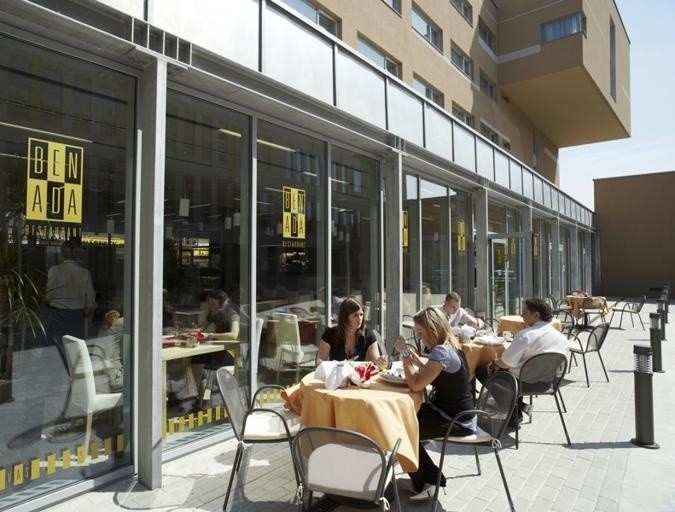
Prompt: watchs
<box><xmin>399</xmin><ymin>349</ymin><xmax>411</xmax><ymax>358</ymax></box>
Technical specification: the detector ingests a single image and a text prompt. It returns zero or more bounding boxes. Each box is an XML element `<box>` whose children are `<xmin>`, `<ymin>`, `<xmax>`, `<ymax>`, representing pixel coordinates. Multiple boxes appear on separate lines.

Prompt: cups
<box><xmin>502</xmin><ymin>330</ymin><xmax>513</xmax><ymax>340</ymax></box>
<box><xmin>465</xmin><ymin>334</ymin><xmax>470</xmax><ymax>343</ymax></box>
<box><xmin>185</xmin><ymin>336</ymin><xmax>197</xmax><ymax>347</ymax></box>
<box><xmin>485</xmin><ymin>326</ymin><xmax>492</xmax><ymax>333</ymax></box>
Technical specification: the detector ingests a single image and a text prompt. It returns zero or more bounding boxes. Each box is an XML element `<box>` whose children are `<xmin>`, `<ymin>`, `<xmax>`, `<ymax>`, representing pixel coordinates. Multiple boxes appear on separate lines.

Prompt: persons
<box><xmin>476</xmin><ymin>297</ymin><xmax>569</xmax><ymax>427</ymax></box>
<box><xmin>318</xmin><ymin>299</ymin><xmax>389</xmax><ymax>368</ymax></box>
<box><xmin>424</xmin><ymin>293</ymin><xmax>484</xmax><ymax>404</ymax></box>
<box><xmin>394</xmin><ymin>307</ymin><xmax>478</xmax><ymax>495</ymax></box>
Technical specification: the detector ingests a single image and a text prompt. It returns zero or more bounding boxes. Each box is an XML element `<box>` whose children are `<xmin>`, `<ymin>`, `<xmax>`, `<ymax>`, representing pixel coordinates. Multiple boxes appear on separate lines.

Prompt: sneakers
<box><xmin>522</xmin><ymin>403</ymin><xmax>533</xmax><ymax>417</ymax></box>
<box><xmin>410</xmin><ymin>484</ymin><xmax>447</xmax><ymax>501</ymax></box>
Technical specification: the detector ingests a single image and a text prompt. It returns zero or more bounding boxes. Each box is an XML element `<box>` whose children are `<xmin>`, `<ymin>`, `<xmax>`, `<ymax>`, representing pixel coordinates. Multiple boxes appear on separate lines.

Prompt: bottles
<box><xmin>584</xmin><ymin>291</ymin><xmax>588</xmax><ymax>297</ymax></box>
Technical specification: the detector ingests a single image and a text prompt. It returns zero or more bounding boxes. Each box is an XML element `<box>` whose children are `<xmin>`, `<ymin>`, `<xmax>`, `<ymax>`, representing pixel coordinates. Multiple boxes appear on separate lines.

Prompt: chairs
<box><xmin>548</xmin><ymin>289</ymin><xmax>647</xmax><ymax>331</ymax></box>
<box><xmin>511</xmin><ymin>352</ymin><xmax>573</xmax><ymax>448</ymax></box>
<box><xmin>56</xmin><ymin>333</ymin><xmax>124</xmax><ymax>452</ymax></box>
<box><xmin>273</xmin><ymin>312</ymin><xmax>318</xmax><ymax>384</ymax></box>
<box><xmin>199</xmin><ymin>317</ymin><xmax>263</xmax><ymax>406</ymax></box>
<box><xmin>215</xmin><ymin>365</ymin><xmax>305</xmax><ymax>511</ymax></box>
<box><xmin>291</xmin><ymin>423</ymin><xmax>406</xmax><ymax>511</ymax></box>
<box><xmin>563</xmin><ymin>321</ymin><xmax>612</xmax><ymax>388</ymax></box>
<box><xmin>427</xmin><ymin>369</ymin><xmax>520</xmax><ymax>511</ymax></box>
<box><xmin>165</xmin><ymin>284</ymin><xmax>432</xmax><ymax>325</ymax></box>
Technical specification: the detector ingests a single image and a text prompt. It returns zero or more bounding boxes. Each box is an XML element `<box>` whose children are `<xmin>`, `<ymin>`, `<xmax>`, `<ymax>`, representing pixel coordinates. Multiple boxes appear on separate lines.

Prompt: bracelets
<box><xmin>492</xmin><ymin>359</ymin><xmax>495</xmax><ymax>365</ymax></box>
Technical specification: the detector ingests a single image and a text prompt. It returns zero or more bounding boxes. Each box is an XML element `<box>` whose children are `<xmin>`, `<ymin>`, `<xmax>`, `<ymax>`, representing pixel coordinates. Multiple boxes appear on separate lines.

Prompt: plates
<box><xmin>353</xmin><ymin>360</ymin><xmax>380</xmax><ymax>376</ymax></box>
<box><xmin>378</xmin><ymin>367</ymin><xmax>406</xmax><ymax>386</ymax></box>
